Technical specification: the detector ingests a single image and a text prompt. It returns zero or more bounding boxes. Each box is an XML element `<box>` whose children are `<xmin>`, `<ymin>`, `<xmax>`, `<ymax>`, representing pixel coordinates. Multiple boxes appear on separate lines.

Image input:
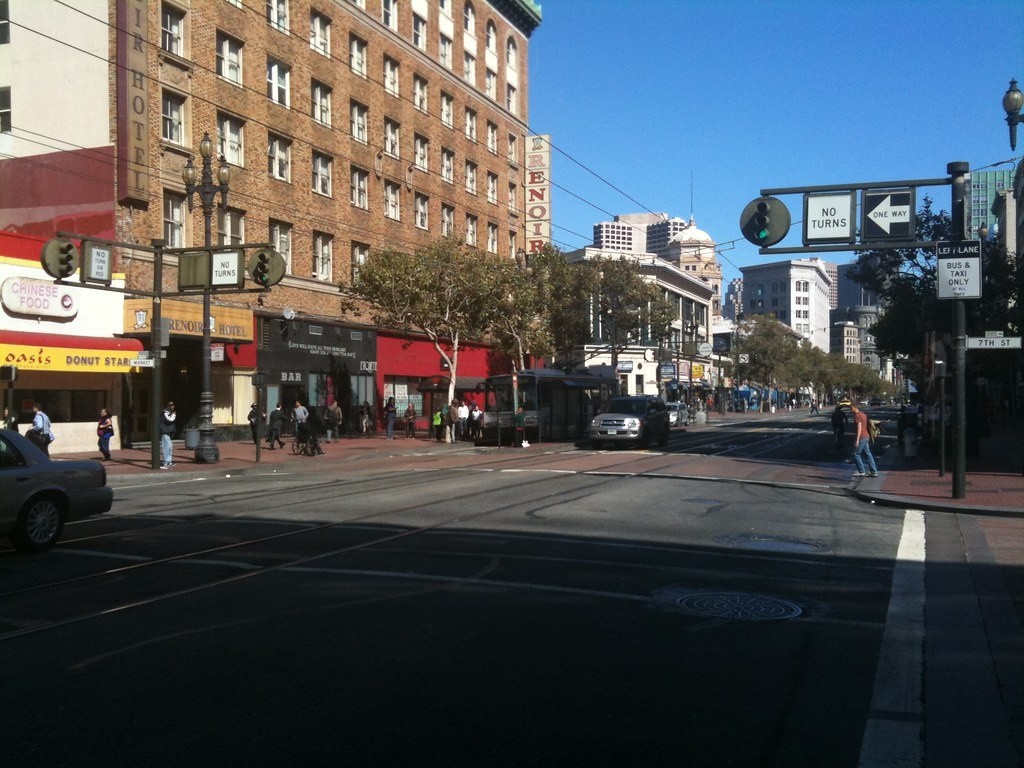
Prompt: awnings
<box><xmin>665</xmin><ymin>381</ymin><xmax>715</xmax><ymax>391</ymax></box>
<box><xmin>0</xmin><ymin>331</ymin><xmax>144</xmax><ymax>373</ymax></box>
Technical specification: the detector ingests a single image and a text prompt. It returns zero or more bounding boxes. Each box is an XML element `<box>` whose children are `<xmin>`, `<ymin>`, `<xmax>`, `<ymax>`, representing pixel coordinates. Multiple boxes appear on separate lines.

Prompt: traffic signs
<box><xmin>965</xmin><ymin>337</ymin><xmax>1022</xmax><ymax>349</ymax></box>
<box><xmin>861</xmin><ymin>192</ymin><xmax>910</xmax><ymax>238</ymax></box>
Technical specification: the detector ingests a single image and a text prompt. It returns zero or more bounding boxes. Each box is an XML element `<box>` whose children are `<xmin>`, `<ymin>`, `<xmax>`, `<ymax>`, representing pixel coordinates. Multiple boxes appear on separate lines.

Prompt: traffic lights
<box><xmin>755</xmin><ymin>202</ymin><xmax>770</xmax><ymax>240</ymax></box>
<box><xmin>258</xmin><ymin>252</ymin><xmax>269</xmax><ymax>284</ymax></box>
<box><xmin>895</xmin><ymin>359</ymin><xmax>900</xmax><ymax>368</ymax></box>
<box><xmin>58</xmin><ymin>243</ymin><xmax>74</xmax><ymax>279</ymax></box>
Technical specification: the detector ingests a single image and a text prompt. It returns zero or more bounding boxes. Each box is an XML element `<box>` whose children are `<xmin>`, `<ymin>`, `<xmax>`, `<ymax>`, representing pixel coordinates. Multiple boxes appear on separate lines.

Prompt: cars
<box><xmin>0</xmin><ymin>426</ymin><xmax>111</xmax><ymax>559</ymax></box>
<box><xmin>861</xmin><ymin>398</ymin><xmax>887</xmax><ymax>406</ymax></box>
<box><xmin>839</xmin><ymin>399</ymin><xmax>853</xmax><ymax>408</ymax></box>
<box><xmin>668</xmin><ymin>403</ymin><xmax>690</xmax><ymax>426</ymax></box>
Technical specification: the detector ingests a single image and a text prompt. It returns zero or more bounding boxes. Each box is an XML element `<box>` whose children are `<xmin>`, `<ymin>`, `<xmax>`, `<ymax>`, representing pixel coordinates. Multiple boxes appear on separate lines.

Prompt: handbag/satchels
<box><xmin>25</xmin><ymin>429</ymin><xmax>50</xmax><ymax>445</ymax></box>
<box><xmin>104</xmin><ymin>425</ymin><xmax>114</xmax><ymax>436</ymax></box>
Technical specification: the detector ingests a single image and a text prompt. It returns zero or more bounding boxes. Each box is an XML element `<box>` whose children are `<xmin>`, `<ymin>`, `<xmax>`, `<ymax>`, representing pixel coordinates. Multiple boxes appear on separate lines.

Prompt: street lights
<box><xmin>685</xmin><ymin>311</ymin><xmax>700</xmax><ymax>403</ymax></box>
<box><xmin>182</xmin><ymin>132</ymin><xmax>232</xmax><ymax>463</ymax></box>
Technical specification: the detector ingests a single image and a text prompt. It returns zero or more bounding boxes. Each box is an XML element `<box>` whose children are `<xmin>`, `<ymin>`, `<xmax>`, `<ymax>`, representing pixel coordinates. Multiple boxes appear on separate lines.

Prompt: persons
<box><xmin>511</xmin><ymin>407</ymin><xmax>525</xmax><ymax>447</ymax></box>
<box><xmin>850</xmin><ymin>404</ymin><xmax>879</xmax><ymax>477</ymax></box>
<box><xmin>431</xmin><ymin>399</ymin><xmax>484</xmax><ymax>443</ymax></box>
<box><xmin>360</xmin><ymin>401</ymin><xmax>375</xmax><ymax>437</ymax></box>
<box><xmin>248</xmin><ymin>403</ymin><xmax>266</xmax><ymax>445</ymax></box>
<box><xmin>323</xmin><ymin>400</ymin><xmax>342</xmax><ymax>443</ymax></box>
<box><xmin>831</xmin><ymin>405</ymin><xmax>848</xmax><ymax>447</ymax></box>
<box><xmin>292</xmin><ymin>400</ymin><xmax>325</xmax><ymax>455</ymax></box>
<box><xmin>385</xmin><ymin>398</ymin><xmax>398</xmax><ymax>440</ymax></box>
<box><xmin>160</xmin><ymin>402</ymin><xmax>176</xmax><ymax>470</ymax></box>
<box><xmin>705</xmin><ymin>395</ymin><xmax>819</xmax><ymax>414</ymax></box>
<box><xmin>97</xmin><ymin>408</ymin><xmax>114</xmax><ymax>461</ymax></box>
<box><xmin>32</xmin><ymin>402</ymin><xmax>53</xmax><ymax>457</ymax></box>
<box><xmin>269</xmin><ymin>402</ymin><xmax>293</xmax><ymax>450</ymax></box>
<box><xmin>405</xmin><ymin>402</ymin><xmax>417</xmax><ymax>440</ymax></box>
<box><xmin>3</xmin><ymin>405</ymin><xmax>19</xmax><ymax>451</ymax></box>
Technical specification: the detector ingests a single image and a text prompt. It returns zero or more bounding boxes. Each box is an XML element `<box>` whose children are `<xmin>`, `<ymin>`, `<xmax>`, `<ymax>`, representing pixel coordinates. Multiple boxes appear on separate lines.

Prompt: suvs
<box><xmin>589</xmin><ymin>393</ymin><xmax>669</xmax><ymax>447</ymax></box>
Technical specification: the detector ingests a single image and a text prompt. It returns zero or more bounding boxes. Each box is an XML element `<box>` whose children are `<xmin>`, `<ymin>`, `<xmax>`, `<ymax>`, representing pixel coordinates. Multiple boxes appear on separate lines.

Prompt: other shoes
<box><xmin>853</xmin><ymin>471</ymin><xmax>878</xmax><ymax>477</ymax></box>
<box><xmin>280</xmin><ymin>443</ymin><xmax>286</xmax><ymax>449</ymax></box>
<box><xmin>269</xmin><ymin>447</ymin><xmax>276</xmax><ymax>450</ymax></box>
<box><xmin>162</xmin><ymin>462</ymin><xmax>176</xmax><ymax>467</ymax></box>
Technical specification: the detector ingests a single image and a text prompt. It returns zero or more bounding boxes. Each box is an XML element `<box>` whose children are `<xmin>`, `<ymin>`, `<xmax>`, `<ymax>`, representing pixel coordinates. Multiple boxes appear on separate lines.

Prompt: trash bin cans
<box><xmin>185</xmin><ymin>428</ymin><xmax>200</xmax><ymax>450</ymax></box>
<box><xmin>898</xmin><ymin>413</ymin><xmax>918</xmax><ymax>447</ymax></box>
<box><xmin>903</xmin><ymin>428</ymin><xmax>918</xmax><ymax>460</ymax></box>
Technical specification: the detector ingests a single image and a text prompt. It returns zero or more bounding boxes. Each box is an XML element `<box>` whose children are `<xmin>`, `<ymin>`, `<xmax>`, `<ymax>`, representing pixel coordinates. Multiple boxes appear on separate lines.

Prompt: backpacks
<box><xmin>327</xmin><ymin>406</ymin><xmax>339</xmax><ymax>423</ymax></box>
<box><xmin>382</xmin><ymin>406</ymin><xmax>387</xmax><ymax>419</ymax></box>
<box><xmin>867</xmin><ymin>417</ymin><xmax>880</xmax><ymax>438</ymax></box>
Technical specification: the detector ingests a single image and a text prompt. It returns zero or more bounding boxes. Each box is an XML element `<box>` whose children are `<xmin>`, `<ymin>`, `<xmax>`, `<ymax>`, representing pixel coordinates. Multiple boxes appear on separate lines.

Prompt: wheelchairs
<box><xmin>292</xmin><ymin>419</ymin><xmax>315</xmax><ymax>457</ymax></box>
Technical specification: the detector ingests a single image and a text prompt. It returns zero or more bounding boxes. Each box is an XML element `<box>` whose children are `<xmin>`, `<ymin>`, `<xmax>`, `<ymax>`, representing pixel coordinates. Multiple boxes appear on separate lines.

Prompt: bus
<box><xmin>476</xmin><ymin>369</ymin><xmax>620</xmax><ymax>442</ymax></box>
